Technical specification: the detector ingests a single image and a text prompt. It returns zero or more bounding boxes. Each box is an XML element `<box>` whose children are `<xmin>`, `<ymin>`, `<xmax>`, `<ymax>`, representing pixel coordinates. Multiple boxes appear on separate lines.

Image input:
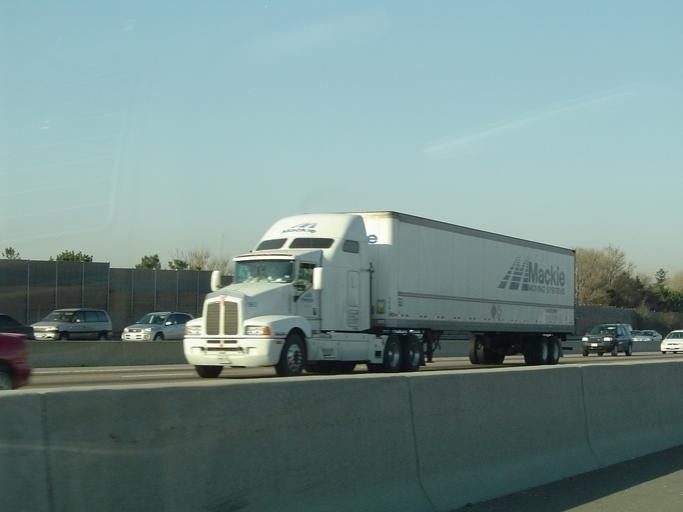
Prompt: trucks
<box><xmin>183</xmin><ymin>211</ymin><xmax>576</xmax><ymax>378</ymax></box>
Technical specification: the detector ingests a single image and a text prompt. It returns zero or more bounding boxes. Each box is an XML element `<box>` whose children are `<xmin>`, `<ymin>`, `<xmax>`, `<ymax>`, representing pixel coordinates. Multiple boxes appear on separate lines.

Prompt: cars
<box><xmin>0</xmin><ymin>333</ymin><xmax>31</xmax><ymax>390</ymax></box>
<box><xmin>582</xmin><ymin>324</ymin><xmax>632</xmax><ymax>356</ymax></box>
<box><xmin>661</xmin><ymin>330</ymin><xmax>683</xmax><ymax>354</ymax></box>
<box><xmin>632</xmin><ymin>330</ymin><xmax>662</xmax><ymax>341</ymax></box>
<box><xmin>0</xmin><ymin>308</ymin><xmax>192</xmax><ymax>341</ymax></box>
